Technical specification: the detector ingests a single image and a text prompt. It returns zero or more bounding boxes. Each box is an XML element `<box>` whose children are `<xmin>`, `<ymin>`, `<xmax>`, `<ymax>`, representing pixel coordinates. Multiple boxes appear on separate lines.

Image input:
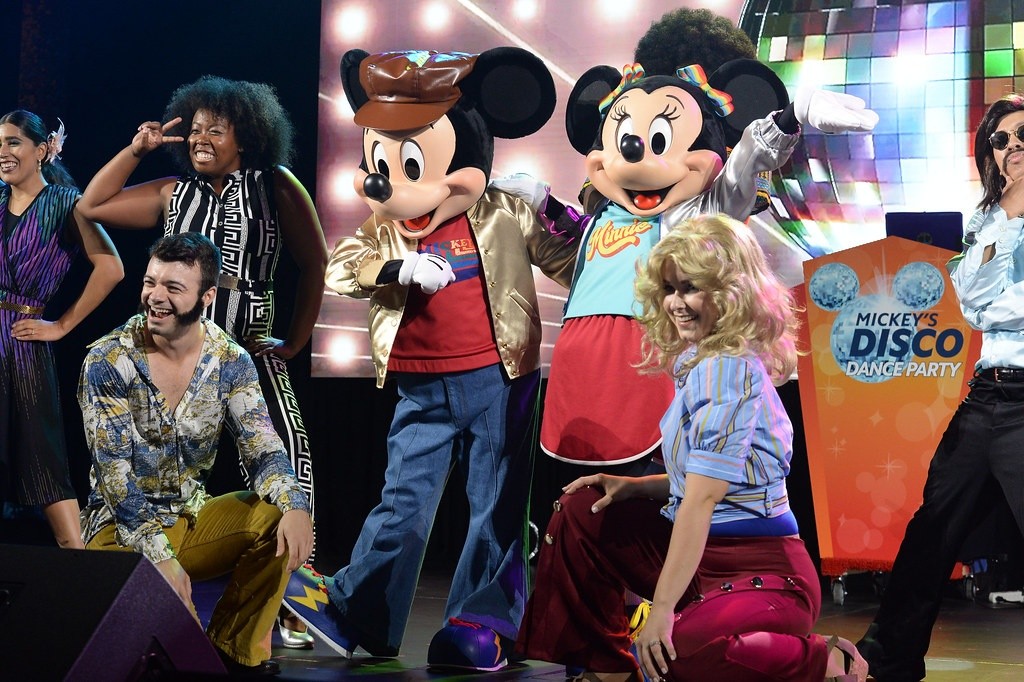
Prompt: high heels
<box><xmin>276</xmin><ymin>613</ymin><xmax>315</xmax><ymax>649</ymax></box>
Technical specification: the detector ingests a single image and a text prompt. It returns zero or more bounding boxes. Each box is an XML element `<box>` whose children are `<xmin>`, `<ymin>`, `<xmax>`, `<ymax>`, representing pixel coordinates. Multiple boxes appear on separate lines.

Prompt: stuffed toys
<box><xmin>283</xmin><ymin>46</ymin><xmax>579</xmax><ymax>672</ymax></box>
<box><xmin>491</xmin><ymin>6</ymin><xmax>881</xmax><ymax>682</ymax></box>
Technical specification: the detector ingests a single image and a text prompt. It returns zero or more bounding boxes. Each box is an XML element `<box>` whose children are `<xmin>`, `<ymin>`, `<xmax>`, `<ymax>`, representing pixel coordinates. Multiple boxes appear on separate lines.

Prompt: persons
<box><xmin>511</xmin><ymin>213</ymin><xmax>869</xmax><ymax>682</ymax></box>
<box><xmin>856</xmin><ymin>93</ymin><xmax>1024</xmax><ymax>682</ymax></box>
<box><xmin>0</xmin><ymin>75</ymin><xmax>329</xmax><ymax>677</ymax></box>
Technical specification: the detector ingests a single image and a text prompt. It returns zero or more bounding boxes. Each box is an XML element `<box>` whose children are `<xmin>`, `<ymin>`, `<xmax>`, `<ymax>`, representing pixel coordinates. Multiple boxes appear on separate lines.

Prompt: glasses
<box><xmin>988</xmin><ymin>125</ymin><xmax>1024</xmax><ymax>150</ymax></box>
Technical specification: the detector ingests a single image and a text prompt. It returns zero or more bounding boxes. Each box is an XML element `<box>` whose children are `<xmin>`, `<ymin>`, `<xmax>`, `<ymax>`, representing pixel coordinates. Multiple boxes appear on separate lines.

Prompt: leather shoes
<box><xmin>213</xmin><ymin>642</ymin><xmax>281</xmax><ymax>677</ymax></box>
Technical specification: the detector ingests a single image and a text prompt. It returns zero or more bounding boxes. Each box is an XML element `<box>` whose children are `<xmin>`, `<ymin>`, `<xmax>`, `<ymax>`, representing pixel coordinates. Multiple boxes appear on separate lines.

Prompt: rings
<box><xmin>32</xmin><ymin>328</ymin><xmax>34</xmax><ymax>335</ymax></box>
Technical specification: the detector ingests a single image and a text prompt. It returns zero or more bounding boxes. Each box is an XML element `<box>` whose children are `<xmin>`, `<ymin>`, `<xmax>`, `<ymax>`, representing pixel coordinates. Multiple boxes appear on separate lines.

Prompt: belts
<box><xmin>217</xmin><ymin>273</ymin><xmax>274</xmax><ymax>292</ymax></box>
<box><xmin>981</xmin><ymin>366</ymin><xmax>1024</xmax><ymax>382</ymax></box>
<box><xmin>0</xmin><ymin>301</ymin><xmax>45</xmax><ymax>314</ymax></box>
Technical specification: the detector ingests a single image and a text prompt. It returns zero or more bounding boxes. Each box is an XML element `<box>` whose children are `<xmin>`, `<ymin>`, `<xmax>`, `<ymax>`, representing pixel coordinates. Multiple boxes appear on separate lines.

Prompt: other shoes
<box><xmin>850</xmin><ymin>645</ymin><xmax>927</xmax><ymax>682</ymax></box>
<box><xmin>580</xmin><ymin>670</ymin><xmax>645</xmax><ymax>682</ymax></box>
<box><xmin>820</xmin><ymin>633</ymin><xmax>869</xmax><ymax>682</ymax></box>
